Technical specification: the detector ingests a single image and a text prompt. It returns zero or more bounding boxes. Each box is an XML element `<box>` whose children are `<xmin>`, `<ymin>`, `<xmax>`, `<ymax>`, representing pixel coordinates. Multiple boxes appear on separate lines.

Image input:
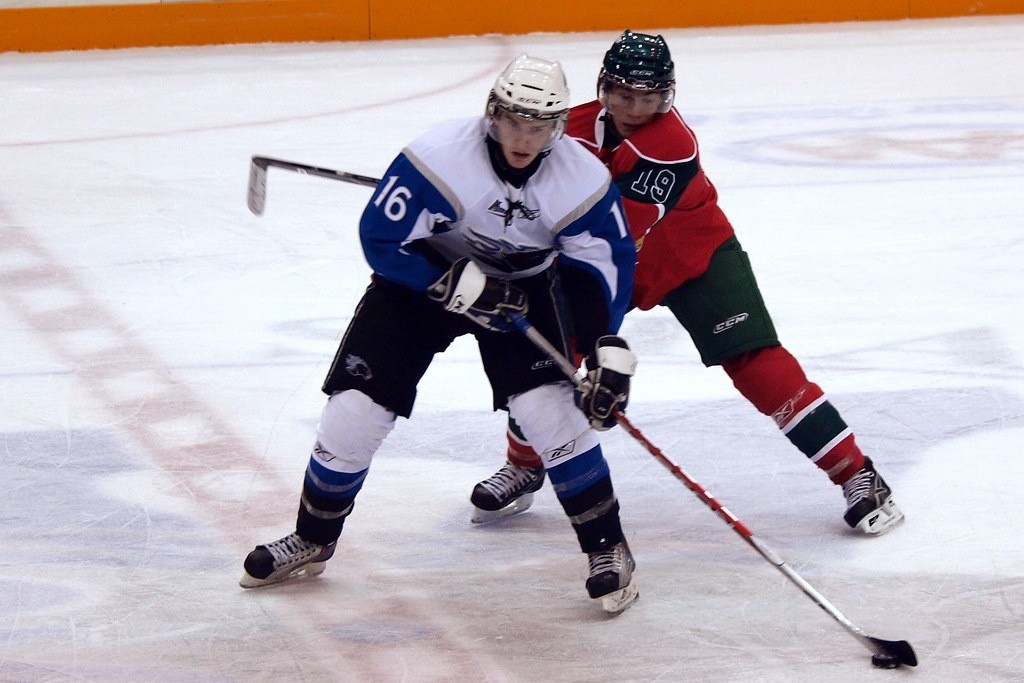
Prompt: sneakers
<box><xmin>584</xmin><ymin>536</ymin><xmax>639</xmax><ymax>614</ymax></box>
<box><xmin>841</xmin><ymin>456</ymin><xmax>903</xmax><ymax>534</ymax></box>
<box><xmin>240</xmin><ymin>533</ymin><xmax>338</xmax><ymax>589</ymax></box>
<box><xmin>470</xmin><ymin>460</ymin><xmax>545</xmax><ymax>525</ymax></box>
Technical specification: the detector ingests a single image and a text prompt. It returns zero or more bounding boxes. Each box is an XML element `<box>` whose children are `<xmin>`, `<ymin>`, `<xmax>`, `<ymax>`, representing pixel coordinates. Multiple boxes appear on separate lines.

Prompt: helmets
<box><xmin>490</xmin><ymin>53</ymin><xmax>569</xmax><ymax>137</ymax></box>
<box><xmin>596</xmin><ymin>29</ymin><xmax>675</xmax><ymax>107</ymax></box>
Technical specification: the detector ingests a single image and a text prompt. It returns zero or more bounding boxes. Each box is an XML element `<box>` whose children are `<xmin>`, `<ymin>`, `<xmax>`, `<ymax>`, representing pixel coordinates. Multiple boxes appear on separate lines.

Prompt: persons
<box><xmin>240</xmin><ymin>53</ymin><xmax>641</xmax><ymax>615</ymax></box>
<box><xmin>469</xmin><ymin>30</ymin><xmax>906</xmax><ymax>537</ymax></box>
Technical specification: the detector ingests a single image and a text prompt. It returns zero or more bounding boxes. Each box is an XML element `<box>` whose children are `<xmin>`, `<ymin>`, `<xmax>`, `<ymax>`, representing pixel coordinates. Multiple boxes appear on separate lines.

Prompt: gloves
<box><xmin>574</xmin><ymin>334</ymin><xmax>638</xmax><ymax>431</ymax></box>
<box><xmin>429</xmin><ymin>258</ymin><xmax>526</xmax><ymax>332</ymax></box>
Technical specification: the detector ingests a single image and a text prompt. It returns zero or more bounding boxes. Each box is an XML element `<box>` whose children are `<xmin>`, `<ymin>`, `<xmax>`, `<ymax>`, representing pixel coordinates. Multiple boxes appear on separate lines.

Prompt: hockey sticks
<box><xmin>243</xmin><ymin>154</ymin><xmax>382</xmax><ymax>218</ymax></box>
<box><xmin>502</xmin><ymin>308</ymin><xmax>920</xmax><ymax>672</ymax></box>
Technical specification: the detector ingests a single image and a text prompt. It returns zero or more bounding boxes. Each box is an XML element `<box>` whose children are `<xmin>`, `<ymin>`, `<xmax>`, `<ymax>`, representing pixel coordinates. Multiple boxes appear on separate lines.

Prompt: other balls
<box><xmin>871</xmin><ymin>651</ymin><xmax>901</xmax><ymax>669</ymax></box>
